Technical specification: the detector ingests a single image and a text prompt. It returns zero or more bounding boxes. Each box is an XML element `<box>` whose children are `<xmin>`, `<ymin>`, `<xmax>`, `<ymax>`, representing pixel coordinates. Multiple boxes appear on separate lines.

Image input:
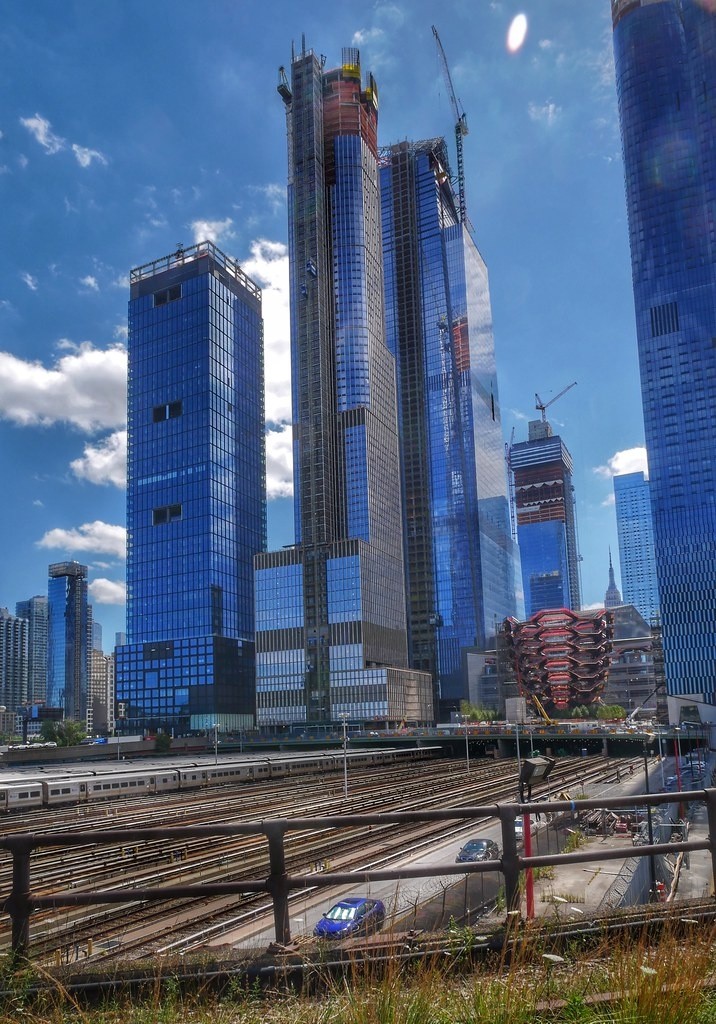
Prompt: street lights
<box><xmin>116</xmin><ymin>729</ymin><xmax>121</xmax><ymax>764</ymax></box>
<box><xmin>338</xmin><ymin>712</ymin><xmax>351</xmax><ymax>797</ymax></box>
<box><xmin>213</xmin><ymin>723</ymin><xmax>220</xmax><ymax>769</ymax></box>
<box><xmin>462</xmin><ymin>714</ymin><xmax>470</xmax><ymax>775</ymax></box>
<box><xmin>652</xmin><ymin>717</ymin><xmax>665</xmax><ymax>787</ymax></box>
<box><xmin>675</xmin><ymin>728</ymin><xmax>681</xmax><ymax>769</ymax></box>
<box><xmin>453</xmin><ymin>706</ymin><xmax>458</xmax><ymax>725</ymax></box>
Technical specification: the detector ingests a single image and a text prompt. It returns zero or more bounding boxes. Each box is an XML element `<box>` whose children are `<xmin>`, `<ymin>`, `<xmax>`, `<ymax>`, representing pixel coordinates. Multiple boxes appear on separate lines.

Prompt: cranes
<box><xmin>432</xmin><ymin>27</ymin><xmax>471</xmax><ymax>224</ymax></box>
<box><xmin>536</xmin><ymin>381</ymin><xmax>576</xmax><ymax>421</ymax></box>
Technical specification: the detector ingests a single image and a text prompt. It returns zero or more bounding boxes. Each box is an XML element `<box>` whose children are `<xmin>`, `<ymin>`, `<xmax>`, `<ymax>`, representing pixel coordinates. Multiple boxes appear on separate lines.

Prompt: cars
<box><xmin>515</xmin><ymin>819</ymin><xmax>539</xmax><ymax>840</ymax></box>
<box><xmin>456</xmin><ymin>839</ymin><xmax>500</xmax><ymax>862</ymax></box>
<box><xmin>11</xmin><ymin>742</ymin><xmax>58</xmax><ymax>749</ymax></box>
<box><xmin>314</xmin><ymin>897</ymin><xmax>386</xmax><ymax>941</ymax></box>
